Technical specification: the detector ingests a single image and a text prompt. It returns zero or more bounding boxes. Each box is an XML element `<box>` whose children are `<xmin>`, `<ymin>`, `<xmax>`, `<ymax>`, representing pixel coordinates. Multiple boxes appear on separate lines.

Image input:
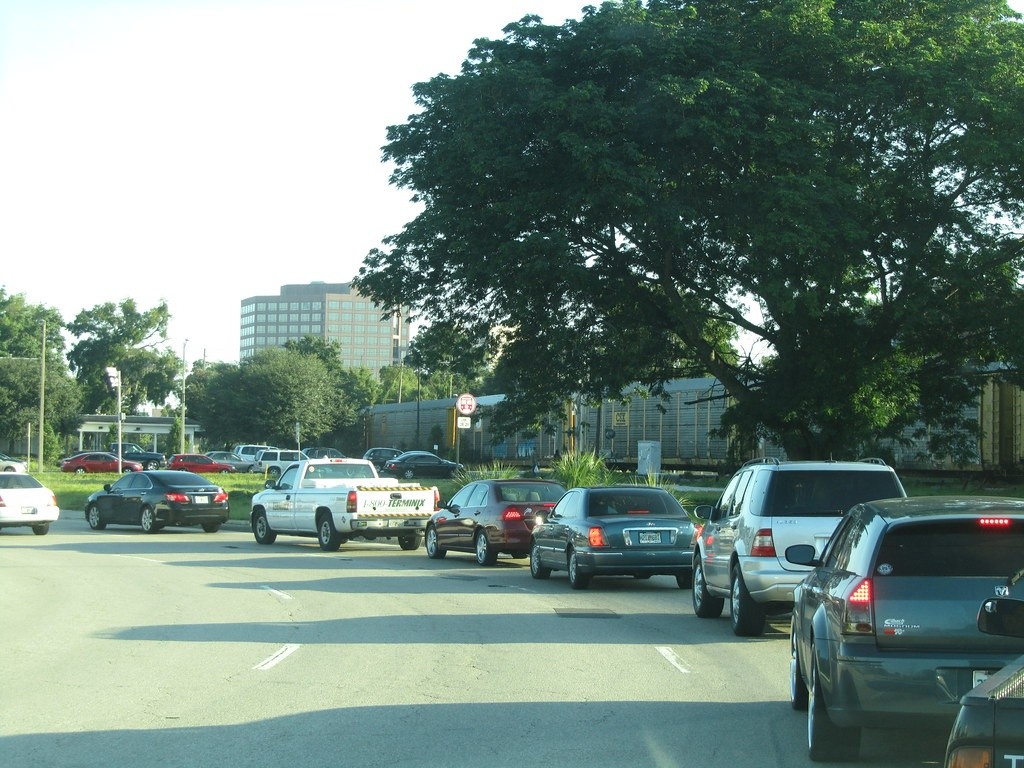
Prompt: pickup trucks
<box><xmin>249</xmin><ymin>458</ymin><xmax>441</xmax><ymax>552</ymax></box>
<box><xmin>74</xmin><ymin>443</ymin><xmax>167</xmax><ymax>471</ymax></box>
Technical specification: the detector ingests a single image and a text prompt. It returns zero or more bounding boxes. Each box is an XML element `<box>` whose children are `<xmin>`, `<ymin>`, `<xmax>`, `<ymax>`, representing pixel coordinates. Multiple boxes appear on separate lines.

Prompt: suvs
<box><xmin>691</xmin><ymin>456</ymin><xmax>907</xmax><ymax>637</ymax></box>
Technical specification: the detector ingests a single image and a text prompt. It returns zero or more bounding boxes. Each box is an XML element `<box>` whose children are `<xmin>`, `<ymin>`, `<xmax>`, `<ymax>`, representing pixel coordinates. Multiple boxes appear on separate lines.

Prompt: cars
<box><xmin>60</xmin><ymin>452</ymin><xmax>143</xmax><ymax>473</ymax></box>
<box><xmin>529</xmin><ymin>487</ymin><xmax>702</xmax><ymax>589</ymax></box>
<box><xmin>83</xmin><ymin>469</ymin><xmax>230</xmax><ymax>534</ymax></box>
<box><xmin>0</xmin><ymin>452</ymin><xmax>59</xmax><ymax>535</ymax></box>
<box><xmin>169</xmin><ymin>450</ymin><xmax>254</xmax><ymax>474</ymax></box>
<box><xmin>363</xmin><ymin>447</ymin><xmax>464</xmax><ymax>479</ymax></box>
<box><xmin>233</xmin><ymin>445</ymin><xmax>350</xmax><ymax>477</ymax></box>
<box><xmin>785</xmin><ymin>496</ymin><xmax>1024</xmax><ymax>768</ymax></box>
<box><xmin>424</xmin><ymin>478</ymin><xmax>569</xmax><ymax>567</ymax></box>
